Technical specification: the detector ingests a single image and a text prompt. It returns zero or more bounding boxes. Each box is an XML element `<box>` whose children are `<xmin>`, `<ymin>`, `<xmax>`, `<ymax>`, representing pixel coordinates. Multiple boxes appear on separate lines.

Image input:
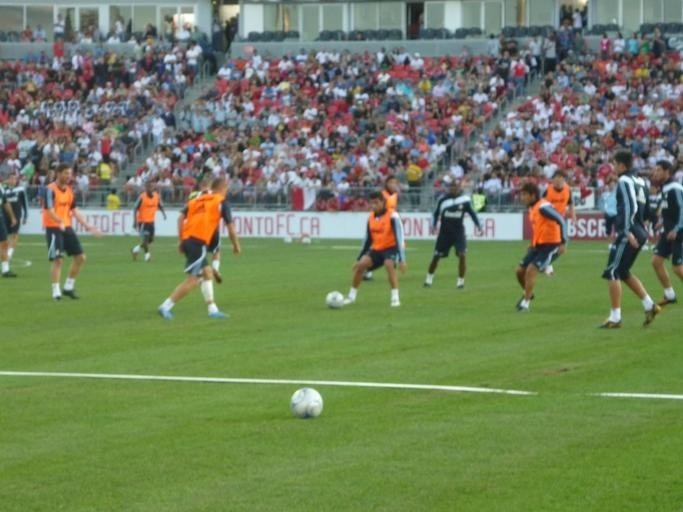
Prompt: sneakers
<box><xmin>657</xmin><ymin>296</ymin><xmax>677</xmax><ymax>305</ymax></box>
<box><xmin>457</xmin><ymin>277</ymin><xmax>464</xmax><ymax>287</ymax></box>
<box><xmin>599</xmin><ymin>318</ymin><xmax>622</xmax><ymax>328</ymax></box>
<box><xmin>52</xmin><ymin>290</ymin><xmax>61</xmax><ymax>299</ymax></box>
<box><xmin>389</xmin><ymin>297</ymin><xmax>400</xmax><ymax>307</ymax></box>
<box><xmin>144</xmin><ymin>251</ymin><xmax>151</xmax><ymax>261</ymax></box>
<box><xmin>3</xmin><ymin>271</ymin><xmax>16</xmax><ymax>278</ymax></box>
<box><xmin>424</xmin><ymin>275</ymin><xmax>434</xmax><ymax>288</ymax></box>
<box><xmin>643</xmin><ymin>303</ymin><xmax>661</xmax><ymax>324</ymax></box>
<box><xmin>158</xmin><ymin>306</ymin><xmax>172</xmax><ymax>319</ymax></box>
<box><xmin>208</xmin><ymin>311</ymin><xmax>226</xmax><ymax>319</ymax></box>
<box><xmin>343</xmin><ymin>297</ymin><xmax>354</xmax><ymax>304</ymax></box>
<box><xmin>131</xmin><ymin>248</ymin><xmax>138</xmax><ymax>261</ymax></box>
<box><xmin>63</xmin><ymin>289</ymin><xmax>79</xmax><ymax>299</ymax></box>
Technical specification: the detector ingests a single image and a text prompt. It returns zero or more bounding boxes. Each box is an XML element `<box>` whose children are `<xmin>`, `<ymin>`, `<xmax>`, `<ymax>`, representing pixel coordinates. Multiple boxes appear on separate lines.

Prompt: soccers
<box><xmin>290</xmin><ymin>388</ymin><xmax>323</xmax><ymax>419</ymax></box>
<box><xmin>283</xmin><ymin>236</ymin><xmax>291</xmax><ymax>243</ymax></box>
<box><xmin>326</xmin><ymin>292</ymin><xmax>345</xmax><ymax>310</ymax></box>
<box><xmin>302</xmin><ymin>237</ymin><xmax>313</xmax><ymax>246</ymax></box>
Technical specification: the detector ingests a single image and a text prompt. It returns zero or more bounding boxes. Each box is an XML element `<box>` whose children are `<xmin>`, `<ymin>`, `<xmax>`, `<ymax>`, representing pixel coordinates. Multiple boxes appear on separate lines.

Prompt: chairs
<box><xmin>0</xmin><ymin>19</ymin><xmax>683</xmax><ymax>213</ymax></box>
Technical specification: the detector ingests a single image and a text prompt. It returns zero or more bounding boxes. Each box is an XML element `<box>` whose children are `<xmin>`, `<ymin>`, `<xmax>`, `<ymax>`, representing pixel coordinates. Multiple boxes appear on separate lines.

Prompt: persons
<box><xmin>422</xmin><ymin>182</ymin><xmax>485</xmax><ymax>289</ymax></box>
<box><xmin>131</xmin><ymin>180</ymin><xmax>167</xmax><ymax>261</ymax></box>
<box><xmin>3</xmin><ymin>175</ymin><xmax>26</xmax><ymax>264</ymax></box>
<box><xmin>649</xmin><ymin>158</ymin><xmax>683</xmax><ymax>305</ymax></box>
<box><xmin>188</xmin><ymin>176</ymin><xmax>223</xmax><ymax>285</ymax></box>
<box><xmin>345</xmin><ymin>191</ymin><xmax>407</xmax><ymax>306</ymax></box>
<box><xmin>0</xmin><ymin>178</ymin><xmax>19</xmax><ymax>278</ymax></box>
<box><xmin>541</xmin><ymin>175</ymin><xmax>575</xmax><ymax>276</ymax></box>
<box><xmin>0</xmin><ymin>1</ymin><xmax>683</xmax><ymax>213</ymax></box>
<box><xmin>39</xmin><ymin>165</ymin><xmax>102</xmax><ymax>301</ymax></box>
<box><xmin>596</xmin><ymin>179</ymin><xmax>619</xmax><ymax>249</ymax></box>
<box><xmin>598</xmin><ymin>150</ymin><xmax>660</xmax><ymax>327</ymax></box>
<box><xmin>156</xmin><ymin>176</ymin><xmax>240</xmax><ymax>321</ymax></box>
<box><xmin>513</xmin><ymin>182</ymin><xmax>568</xmax><ymax>314</ymax></box>
<box><xmin>362</xmin><ymin>176</ymin><xmax>401</xmax><ymax>281</ymax></box>
